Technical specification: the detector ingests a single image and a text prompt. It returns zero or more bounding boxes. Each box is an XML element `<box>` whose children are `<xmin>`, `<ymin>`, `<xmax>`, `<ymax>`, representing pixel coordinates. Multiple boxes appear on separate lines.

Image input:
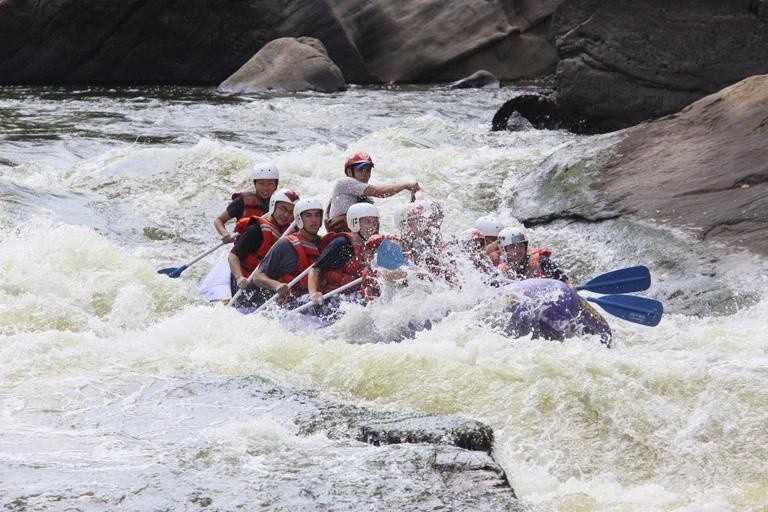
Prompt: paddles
<box><xmin>377</xmin><ymin>240</ymin><xmax>457</xmax><ymax>290</ymax></box>
<box><xmin>158</xmin><ymin>232</ymin><xmax>241</xmax><ymax>278</ymax></box>
<box><xmin>500</xmin><ymin>277</ymin><xmax>662</xmax><ymax>327</ymax></box>
<box><xmin>574</xmin><ymin>266</ymin><xmax>651</xmax><ymax>295</ymax></box>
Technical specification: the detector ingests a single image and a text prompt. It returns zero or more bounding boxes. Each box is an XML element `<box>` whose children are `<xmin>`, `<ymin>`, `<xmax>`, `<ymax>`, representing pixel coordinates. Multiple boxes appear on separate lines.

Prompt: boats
<box><xmin>196</xmin><ymin>244</ymin><xmax>612</xmax><ymax>354</ymax></box>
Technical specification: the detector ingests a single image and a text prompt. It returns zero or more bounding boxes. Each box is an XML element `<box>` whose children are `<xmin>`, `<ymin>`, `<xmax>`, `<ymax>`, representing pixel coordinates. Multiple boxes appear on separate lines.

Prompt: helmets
<box><xmin>268</xmin><ymin>189</ymin><xmax>300</xmax><ymax>215</ymax></box>
<box><xmin>293</xmin><ymin>198</ymin><xmax>323</xmax><ymax>228</ymax></box>
<box><xmin>252</xmin><ymin>162</ymin><xmax>280</xmax><ymax>185</ymax></box>
<box><xmin>395</xmin><ymin>200</ymin><xmax>445</xmax><ymax>229</ymax></box>
<box><xmin>346</xmin><ymin>202</ymin><xmax>380</xmax><ymax>233</ymax></box>
<box><xmin>498</xmin><ymin>227</ymin><xmax>528</xmax><ymax>256</ymax></box>
<box><xmin>344</xmin><ymin>151</ymin><xmax>374</xmax><ymax>175</ymax></box>
<box><xmin>474</xmin><ymin>215</ymin><xmax>507</xmax><ymax>237</ymax></box>
<box><xmin>460</xmin><ymin>229</ymin><xmax>486</xmax><ymax>250</ymax></box>
